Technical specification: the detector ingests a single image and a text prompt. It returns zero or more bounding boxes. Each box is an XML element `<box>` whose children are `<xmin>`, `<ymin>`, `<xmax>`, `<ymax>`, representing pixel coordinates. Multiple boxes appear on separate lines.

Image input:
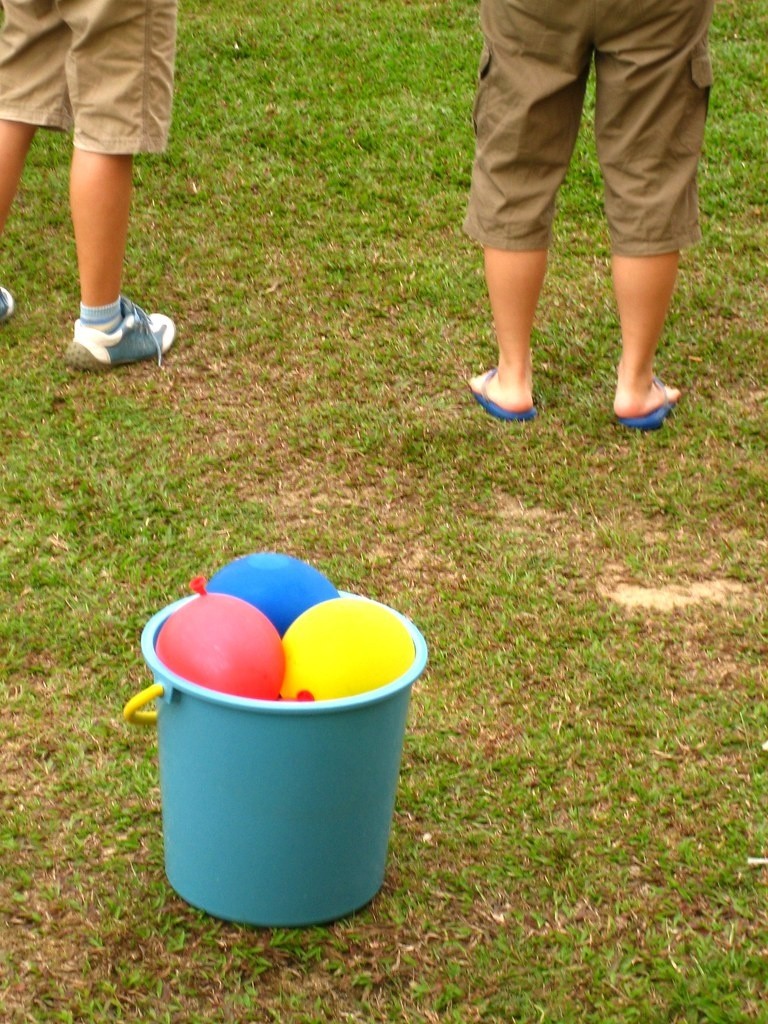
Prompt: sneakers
<box><xmin>68</xmin><ymin>295</ymin><xmax>176</xmax><ymax>368</ymax></box>
<box><xmin>0</xmin><ymin>287</ymin><xmax>14</xmax><ymax>320</ymax></box>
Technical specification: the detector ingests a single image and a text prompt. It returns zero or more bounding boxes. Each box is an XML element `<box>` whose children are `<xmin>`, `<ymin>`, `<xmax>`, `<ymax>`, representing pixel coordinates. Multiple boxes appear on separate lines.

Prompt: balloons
<box><xmin>204</xmin><ymin>552</ymin><xmax>339</xmax><ymax>638</ymax></box>
<box><xmin>153</xmin><ymin>575</ymin><xmax>285</xmax><ymax>699</ymax></box>
<box><xmin>280</xmin><ymin>598</ymin><xmax>415</xmax><ymax>703</ymax></box>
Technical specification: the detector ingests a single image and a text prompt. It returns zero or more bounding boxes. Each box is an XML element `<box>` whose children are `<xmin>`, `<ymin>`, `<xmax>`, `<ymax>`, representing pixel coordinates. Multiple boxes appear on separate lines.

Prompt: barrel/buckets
<box><xmin>124</xmin><ymin>590</ymin><xmax>428</xmax><ymax>926</ymax></box>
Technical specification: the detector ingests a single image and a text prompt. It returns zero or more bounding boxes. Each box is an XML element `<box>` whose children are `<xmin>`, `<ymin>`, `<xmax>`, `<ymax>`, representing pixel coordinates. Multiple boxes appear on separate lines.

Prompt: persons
<box><xmin>0</xmin><ymin>0</ymin><xmax>176</xmax><ymax>371</ymax></box>
<box><xmin>465</xmin><ymin>0</ymin><xmax>715</xmax><ymax>431</ymax></box>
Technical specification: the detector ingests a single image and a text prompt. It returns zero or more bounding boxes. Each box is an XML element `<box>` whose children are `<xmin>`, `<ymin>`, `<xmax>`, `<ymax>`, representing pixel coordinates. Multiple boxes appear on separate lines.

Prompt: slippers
<box><xmin>619</xmin><ymin>377</ymin><xmax>673</xmax><ymax>430</ymax></box>
<box><xmin>468</xmin><ymin>369</ymin><xmax>536</xmax><ymax>421</ymax></box>
